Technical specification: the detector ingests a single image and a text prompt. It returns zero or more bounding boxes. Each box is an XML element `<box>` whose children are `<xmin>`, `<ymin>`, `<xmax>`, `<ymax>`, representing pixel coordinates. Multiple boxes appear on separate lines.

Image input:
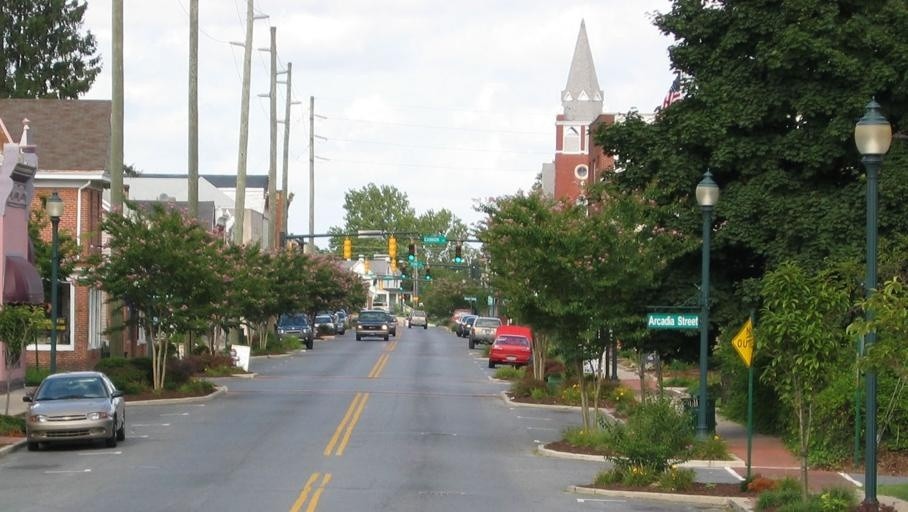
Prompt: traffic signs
<box><xmin>648</xmin><ymin>314</ymin><xmax>698</xmax><ymax>328</ymax></box>
<box><xmin>423</xmin><ymin>236</ymin><xmax>448</xmax><ymax>243</ymax></box>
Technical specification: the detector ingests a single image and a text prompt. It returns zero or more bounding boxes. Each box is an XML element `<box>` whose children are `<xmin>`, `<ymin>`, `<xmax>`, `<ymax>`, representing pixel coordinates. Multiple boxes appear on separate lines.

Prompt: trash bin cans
<box><xmin>682</xmin><ymin>397</ymin><xmax>717</xmax><ymax>432</ymax></box>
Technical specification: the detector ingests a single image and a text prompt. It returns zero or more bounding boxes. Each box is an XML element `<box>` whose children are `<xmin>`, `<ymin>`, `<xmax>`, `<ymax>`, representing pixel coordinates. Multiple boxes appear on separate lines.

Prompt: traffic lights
<box><xmin>401</xmin><ymin>268</ymin><xmax>406</xmax><ymax>279</ymax></box>
<box><xmin>409</xmin><ymin>243</ymin><xmax>415</xmax><ymax>260</ymax></box>
<box><xmin>425</xmin><ymin>269</ymin><xmax>430</xmax><ymax>279</ymax></box>
<box><xmin>455</xmin><ymin>245</ymin><xmax>461</xmax><ymax>263</ymax></box>
<box><xmin>399</xmin><ymin>282</ymin><xmax>403</xmax><ymax>292</ymax></box>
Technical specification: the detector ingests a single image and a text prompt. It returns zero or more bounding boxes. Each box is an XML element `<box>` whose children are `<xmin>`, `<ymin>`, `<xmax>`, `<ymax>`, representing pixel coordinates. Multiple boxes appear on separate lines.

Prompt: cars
<box><xmin>313</xmin><ymin>308</ymin><xmax>348</xmax><ymax>337</ymax></box>
<box><xmin>455</xmin><ymin>313</ymin><xmax>477</xmax><ymax>338</ymax></box>
<box><xmin>468</xmin><ymin>317</ymin><xmax>502</xmax><ymax>349</ymax></box>
<box><xmin>489</xmin><ymin>325</ymin><xmax>538</xmax><ymax>369</ymax></box>
<box><xmin>408</xmin><ymin>309</ymin><xmax>428</xmax><ymax>330</ymax></box>
<box><xmin>23</xmin><ymin>371</ymin><xmax>126</xmax><ymax>451</ymax></box>
<box><xmin>355</xmin><ymin>307</ymin><xmax>397</xmax><ymax>343</ymax></box>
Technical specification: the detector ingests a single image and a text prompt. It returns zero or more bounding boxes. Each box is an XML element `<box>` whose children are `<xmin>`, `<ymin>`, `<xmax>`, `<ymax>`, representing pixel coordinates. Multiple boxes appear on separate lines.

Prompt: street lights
<box><xmin>45</xmin><ymin>189</ymin><xmax>64</xmax><ymax>375</ymax></box>
<box><xmin>853</xmin><ymin>95</ymin><xmax>893</xmax><ymax>510</ymax></box>
<box><xmin>696</xmin><ymin>167</ymin><xmax>720</xmax><ymax>451</ymax></box>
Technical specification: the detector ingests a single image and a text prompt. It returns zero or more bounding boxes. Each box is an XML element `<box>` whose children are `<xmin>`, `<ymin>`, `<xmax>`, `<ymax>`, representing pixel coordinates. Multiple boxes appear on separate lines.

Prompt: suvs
<box><xmin>273</xmin><ymin>312</ymin><xmax>313</xmax><ymax>349</ymax></box>
